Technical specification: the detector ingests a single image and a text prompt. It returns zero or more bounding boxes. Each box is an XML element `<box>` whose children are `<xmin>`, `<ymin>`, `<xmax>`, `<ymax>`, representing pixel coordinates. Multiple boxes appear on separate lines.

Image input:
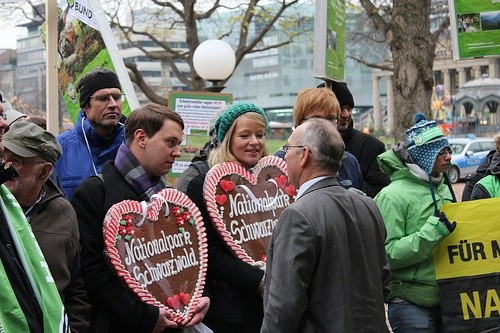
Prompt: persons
<box><xmin>0</xmin><ymin>91</ymin><xmax>73</xmax><ymax>333</ymax></box>
<box><xmin>260</xmin><ymin>118</ymin><xmax>392</xmax><ymax>333</ymax></box>
<box><xmin>461</xmin><ymin>18</ymin><xmax>466</xmax><ymax>32</ymax></box>
<box><xmin>49</xmin><ymin>66</ymin><xmax>128</xmax><ymax>202</ymax></box>
<box><xmin>0</xmin><ymin>121</ymin><xmax>94</xmax><ymax>333</ymax></box>
<box><xmin>470</xmin><ymin>17</ymin><xmax>473</xmax><ymax>26</ymax></box>
<box><xmin>272</xmin><ymin>87</ymin><xmax>373</xmax><ymax>198</ymax></box>
<box><xmin>0</xmin><ymin>91</ymin><xmax>28</xmax><ymax>134</ymax></box>
<box><xmin>373</xmin><ymin>112</ymin><xmax>457</xmax><ymax>333</ymax></box>
<box><xmin>70</xmin><ymin>101</ymin><xmax>213</xmax><ymax>333</ymax></box>
<box><xmin>461</xmin><ymin>132</ymin><xmax>500</xmax><ymax>202</ymax></box>
<box><xmin>316</xmin><ymin>80</ymin><xmax>391</xmax><ymax>199</ymax></box>
<box><xmin>466</xmin><ymin>16</ymin><xmax>470</xmax><ymax>28</ymax></box>
<box><xmin>175</xmin><ymin>100</ymin><xmax>269</xmax><ymax>333</ymax></box>
<box><xmin>458</xmin><ymin>21</ymin><xmax>462</xmax><ymax>33</ymax></box>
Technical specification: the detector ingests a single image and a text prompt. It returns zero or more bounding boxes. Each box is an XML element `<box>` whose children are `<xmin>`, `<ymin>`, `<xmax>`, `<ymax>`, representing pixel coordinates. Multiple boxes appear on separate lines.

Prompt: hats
<box><xmin>317</xmin><ymin>81</ymin><xmax>354</xmax><ymax>109</ymax></box>
<box><xmin>406</xmin><ymin>112</ymin><xmax>457</xmax><ymax>217</ymax></box>
<box><xmin>2</xmin><ymin>119</ymin><xmax>62</xmax><ymax>166</ymax></box>
<box><xmin>215</xmin><ymin>103</ymin><xmax>268</xmax><ymax>143</ymax></box>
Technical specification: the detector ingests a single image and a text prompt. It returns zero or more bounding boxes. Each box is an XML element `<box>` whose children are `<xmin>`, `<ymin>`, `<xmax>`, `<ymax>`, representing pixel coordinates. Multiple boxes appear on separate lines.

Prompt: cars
<box><xmin>443</xmin><ymin>133</ymin><xmax>496</xmax><ymax>183</ymax></box>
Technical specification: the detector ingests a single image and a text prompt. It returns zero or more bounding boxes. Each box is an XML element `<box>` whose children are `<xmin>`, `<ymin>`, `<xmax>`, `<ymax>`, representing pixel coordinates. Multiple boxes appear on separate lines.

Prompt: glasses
<box><xmin>438</xmin><ymin>151</ymin><xmax>452</xmax><ymax>157</ymax></box>
<box><xmin>302</xmin><ymin>115</ymin><xmax>339</xmax><ymax>125</ymax></box>
<box><xmin>0</xmin><ymin>153</ymin><xmax>46</xmax><ymax>170</ymax></box>
<box><xmin>283</xmin><ymin>145</ymin><xmax>312</xmax><ymax>153</ymax></box>
<box><xmin>89</xmin><ymin>94</ymin><xmax>126</xmax><ymax>103</ymax></box>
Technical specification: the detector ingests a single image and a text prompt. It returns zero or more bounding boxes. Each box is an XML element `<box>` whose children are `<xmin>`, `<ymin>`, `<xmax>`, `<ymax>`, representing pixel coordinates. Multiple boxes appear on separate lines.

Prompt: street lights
<box><xmin>192</xmin><ymin>38</ymin><xmax>238</xmax><ymax>91</ymax></box>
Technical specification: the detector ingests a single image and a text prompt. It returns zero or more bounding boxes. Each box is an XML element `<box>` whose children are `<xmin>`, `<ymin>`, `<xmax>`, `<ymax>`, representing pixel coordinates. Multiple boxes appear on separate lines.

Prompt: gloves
<box><xmin>434</xmin><ymin>211</ymin><xmax>457</xmax><ymax>233</ymax></box>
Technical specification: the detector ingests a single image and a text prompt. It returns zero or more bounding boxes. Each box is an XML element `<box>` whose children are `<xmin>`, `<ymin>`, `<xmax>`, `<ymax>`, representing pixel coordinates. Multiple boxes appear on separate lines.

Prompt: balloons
<box><xmin>433</xmin><ymin>100</ymin><xmax>441</xmax><ymax>110</ymax></box>
<box><xmin>446</xmin><ymin>112</ymin><xmax>453</xmax><ymax>121</ymax></box>
<box><xmin>435</xmin><ymin>85</ymin><xmax>445</xmax><ymax>96</ymax></box>
